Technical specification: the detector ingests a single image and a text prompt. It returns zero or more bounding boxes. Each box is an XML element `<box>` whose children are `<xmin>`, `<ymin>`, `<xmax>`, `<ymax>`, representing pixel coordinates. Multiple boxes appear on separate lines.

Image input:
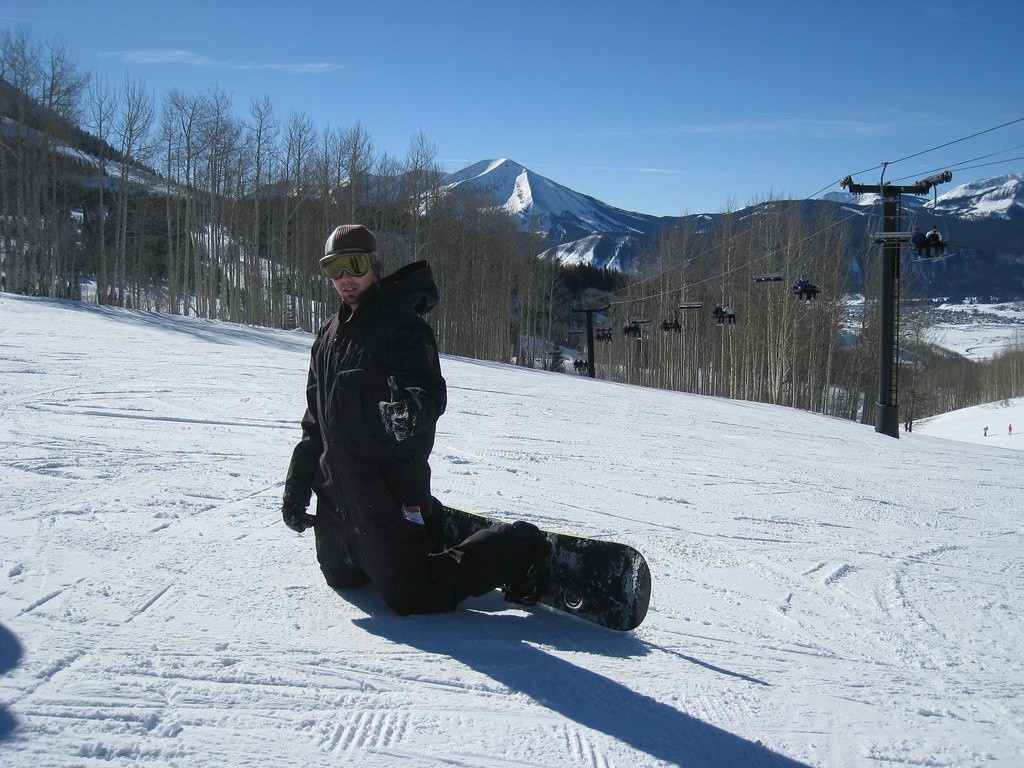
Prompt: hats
<box><xmin>319</xmin><ymin>225</ymin><xmax>377</xmax><ymax>262</ymax></box>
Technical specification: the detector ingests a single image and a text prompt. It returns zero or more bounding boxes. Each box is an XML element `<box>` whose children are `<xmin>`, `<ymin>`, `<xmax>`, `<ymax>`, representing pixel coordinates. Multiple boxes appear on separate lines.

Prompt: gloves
<box><xmin>379</xmin><ymin>376</ymin><xmax>428</xmax><ymax>441</ymax></box>
<box><xmin>282</xmin><ymin>493</ymin><xmax>316</xmax><ymax>532</ymax></box>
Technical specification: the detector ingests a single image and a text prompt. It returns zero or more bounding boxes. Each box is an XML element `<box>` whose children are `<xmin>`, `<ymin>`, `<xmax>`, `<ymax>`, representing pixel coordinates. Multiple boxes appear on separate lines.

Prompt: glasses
<box><xmin>320</xmin><ymin>252</ymin><xmax>377</xmax><ymax>280</ymax></box>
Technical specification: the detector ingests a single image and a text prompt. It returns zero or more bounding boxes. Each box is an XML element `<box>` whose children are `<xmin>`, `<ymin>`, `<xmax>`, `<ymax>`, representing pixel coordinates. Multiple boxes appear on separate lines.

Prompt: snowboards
<box><xmin>432</xmin><ymin>498</ymin><xmax>655</xmax><ymax>634</ymax></box>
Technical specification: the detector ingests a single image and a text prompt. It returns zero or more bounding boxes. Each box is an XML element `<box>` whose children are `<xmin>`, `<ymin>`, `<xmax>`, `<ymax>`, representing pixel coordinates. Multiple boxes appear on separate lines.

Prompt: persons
<box><xmin>712</xmin><ymin>303</ymin><xmax>726</xmax><ymax>327</ymax></box>
<box><xmin>911</xmin><ymin>225</ymin><xmax>930</xmax><ymax>260</ymax></box>
<box><xmin>572</xmin><ymin>357</ymin><xmax>589</xmax><ymax>373</ymax></box>
<box><xmin>280</xmin><ymin>224</ymin><xmax>544</xmax><ymax>616</ymax></box>
<box><xmin>663</xmin><ymin>319</ymin><xmax>669</xmax><ymax>332</ymax></box>
<box><xmin>926</xmin><ymin>225</ymin><xmax>943</xmax><ymax>258</ymax></box>
<box><xmin>797</xmin><ymin>274</ymin><xmax>813</xmax><ymax>305</ymax></box>
<box><xmin>622</xmin><ymin>323</ymin><xmax>642</xmax><ymax>341</ymax></box>
<box><xmin>722</xmin><ymin>305</ymin><xmax>737</xmax><ymax>326</ymax></box>
<box><xmin>674</xmin><ymin>318</ymin><xmax>681</xmax><ymax>332</ymax></box>
<box><xmin>595</xmin><ymin>330</ymin><xmax>613</xmax><ymax>344</ymax></box>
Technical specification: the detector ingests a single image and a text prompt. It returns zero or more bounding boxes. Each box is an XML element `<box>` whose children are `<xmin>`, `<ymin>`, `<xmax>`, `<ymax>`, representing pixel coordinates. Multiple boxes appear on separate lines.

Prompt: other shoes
<box><xmin>504</xmin><ymin>520</ymin><xmax>545</xmax><ymax>605</ymax></box>
